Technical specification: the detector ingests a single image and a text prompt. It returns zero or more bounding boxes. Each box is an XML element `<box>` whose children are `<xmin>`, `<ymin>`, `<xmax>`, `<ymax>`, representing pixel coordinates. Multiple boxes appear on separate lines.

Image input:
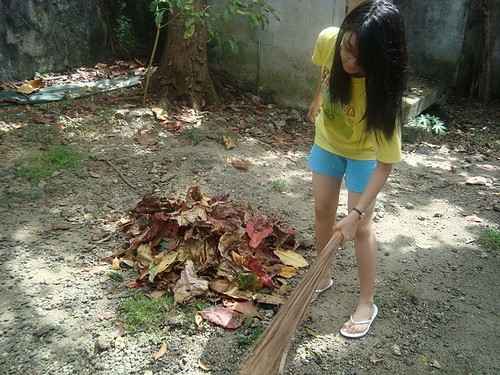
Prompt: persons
<box><xmin>306</xmin><ymin>0</ymin><xmax>411</xmax><ymax>337</ymax></box>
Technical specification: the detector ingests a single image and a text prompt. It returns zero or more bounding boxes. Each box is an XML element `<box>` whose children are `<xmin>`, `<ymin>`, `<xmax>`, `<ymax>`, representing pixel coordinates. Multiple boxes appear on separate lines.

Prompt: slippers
<box><xmin>339</xmin><ymin>303</ymin><xmax>378</xmax><ymax>338</ymax></box>
<box><xmin>309</xmin><ymin>278</ymin><xmax>334</xmax><ymax>304</ymax></box>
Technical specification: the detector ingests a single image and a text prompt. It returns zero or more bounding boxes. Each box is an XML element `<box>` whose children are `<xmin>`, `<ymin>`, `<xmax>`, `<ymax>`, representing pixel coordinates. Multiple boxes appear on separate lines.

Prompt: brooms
<box><xmin>233</xmin><ymin>231</ymin><xmax>344</xmax><ymax>375</ymax></box>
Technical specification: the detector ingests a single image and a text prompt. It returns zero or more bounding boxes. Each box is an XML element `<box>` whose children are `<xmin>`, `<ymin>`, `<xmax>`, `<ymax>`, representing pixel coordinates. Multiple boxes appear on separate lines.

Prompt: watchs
<box><xmin>352</xmin><ymin>208</ymin><xmax>367</xmax><ymax>220</ymax></box>
